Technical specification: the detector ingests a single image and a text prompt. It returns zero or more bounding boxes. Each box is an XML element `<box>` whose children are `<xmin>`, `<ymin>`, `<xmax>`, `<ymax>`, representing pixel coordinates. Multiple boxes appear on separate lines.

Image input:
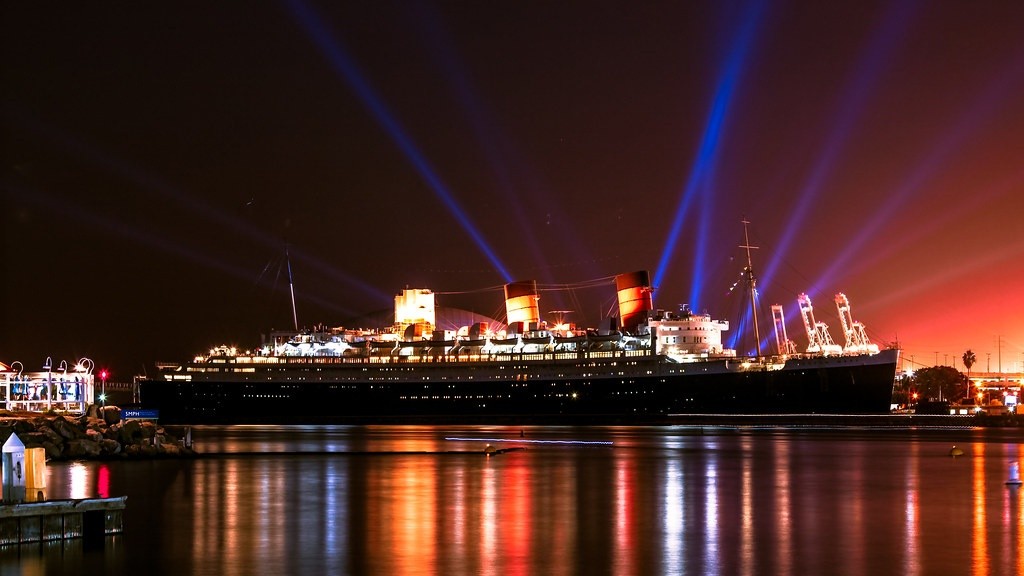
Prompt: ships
<box><xmin>131</xmin><ymin>236</ymin><xmax>900</xmax><ymax>430</ymax></box>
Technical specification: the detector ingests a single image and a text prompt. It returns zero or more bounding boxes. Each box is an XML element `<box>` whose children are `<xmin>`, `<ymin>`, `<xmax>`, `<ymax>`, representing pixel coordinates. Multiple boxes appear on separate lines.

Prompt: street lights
<box><xmin>79</xmin><ymin>359</ymin><xmax>95</xmax><ymax>389</ymax></box>
<box><xmin>42</xmin><ymin>357</ymin><xmax>52</xmax><ymax>410</ymax></box>
<box><xmin>74</xmin><ymin>358</ymin><xmax>90</xmax><ymax>403</ymax></box>
<box><xmin>56</xmin><ymin>360</ymin><xmax>67</xmax><ymax>412</ymax></box>
